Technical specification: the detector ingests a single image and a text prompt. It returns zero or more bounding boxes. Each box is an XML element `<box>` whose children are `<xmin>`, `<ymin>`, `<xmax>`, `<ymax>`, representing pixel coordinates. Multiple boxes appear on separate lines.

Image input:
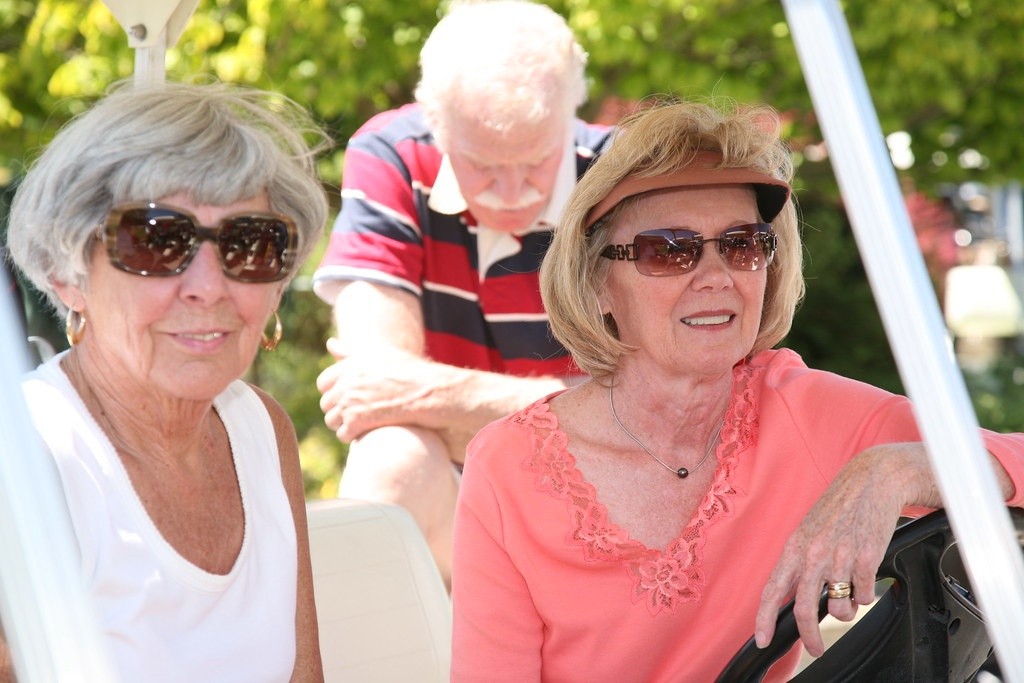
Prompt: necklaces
<box><xmin>608</xmin><ymin>366</ymin><xmax>727</xmax><ymax>480</ymax></box>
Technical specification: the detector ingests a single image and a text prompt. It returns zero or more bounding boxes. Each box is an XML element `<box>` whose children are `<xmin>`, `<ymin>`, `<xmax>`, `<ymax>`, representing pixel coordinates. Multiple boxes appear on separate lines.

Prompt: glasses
<box><xmin>91</xmin><ymin>198</ymin><xmax>298</xmax><ymax>285</ymax></box>
<box><xmin>601</xmin><ymin>223</ymin><xmax>778</xmax><ymax>276</ymax></box>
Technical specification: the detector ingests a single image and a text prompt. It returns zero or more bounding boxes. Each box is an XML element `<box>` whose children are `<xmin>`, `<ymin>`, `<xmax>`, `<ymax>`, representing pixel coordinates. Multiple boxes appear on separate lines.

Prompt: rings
<box><xmin>827</xmin><ymin>581</ymin><xmax>852</xmax><ymax>600</ymax></box>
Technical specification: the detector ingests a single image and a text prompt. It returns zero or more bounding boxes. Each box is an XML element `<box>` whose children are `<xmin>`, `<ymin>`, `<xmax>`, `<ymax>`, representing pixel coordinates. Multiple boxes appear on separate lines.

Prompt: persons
<box><xmin>449</xmin><ymin>99</ymin><xmax>1023</xmax><ymax>682</ymax></box>
<box><xmin>897</xmin><ymin>172</ymin><xmax>1014</xmax><ymax>395</ymax></box>
<box><xmin>0</xmin><ymin>93</ymin><xmax>323</xmax><ymax>683</ymax></box>
<box><xmin>315</xmin><ymin>1</ymin><xmax>627</xmax><ymax>597</ymax></box>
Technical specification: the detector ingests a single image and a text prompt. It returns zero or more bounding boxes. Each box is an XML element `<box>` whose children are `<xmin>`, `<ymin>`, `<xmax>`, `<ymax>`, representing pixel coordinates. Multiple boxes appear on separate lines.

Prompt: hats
<box><xmin>583</xmin><ymin>145</ymin><xmax>791</xmax><ymax>222</ymax></box>
